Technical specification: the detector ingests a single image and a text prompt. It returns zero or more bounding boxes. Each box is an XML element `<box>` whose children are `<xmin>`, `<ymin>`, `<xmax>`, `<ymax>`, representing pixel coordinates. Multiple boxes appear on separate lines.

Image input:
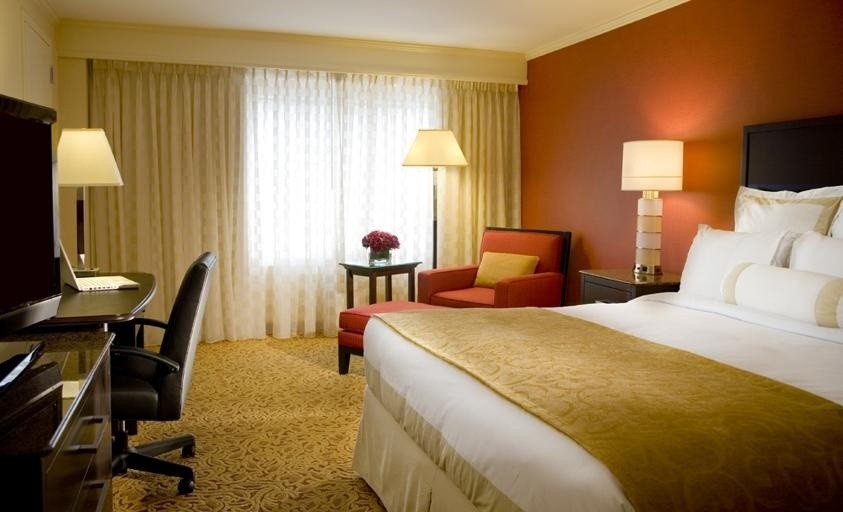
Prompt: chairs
<box><xmin>417</xmin><ymin>226</ymin><xmax>573</xmax><ymax>308</ymax></box>
<box><xmin>107</xmin><ymin>248</ymin><xmax>217</xmax><ymax>489</ymax></box>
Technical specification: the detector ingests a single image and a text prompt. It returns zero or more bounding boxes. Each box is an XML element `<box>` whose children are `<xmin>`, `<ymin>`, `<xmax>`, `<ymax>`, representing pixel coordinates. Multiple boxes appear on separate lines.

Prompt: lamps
<box><xmin>401</xmin><ymin>125</ymin><xmax>468</xmax><ymax>269</ymax></box>
<box><xmin>56</xmin><ymin>125</ymin><xmax>125</xmax><ymax>275</ymax></box>
<box><xmin>620</xmin><ymin>138</ymin><xmax>685</xmax><ymax>277</ymax></box>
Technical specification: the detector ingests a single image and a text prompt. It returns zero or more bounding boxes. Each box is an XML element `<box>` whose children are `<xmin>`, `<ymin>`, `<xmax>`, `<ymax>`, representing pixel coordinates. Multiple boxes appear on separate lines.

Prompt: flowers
<box><xmin>361</xmin><ymin>229</ymin><xmax>399</xmax><ymax>258</ymax></box>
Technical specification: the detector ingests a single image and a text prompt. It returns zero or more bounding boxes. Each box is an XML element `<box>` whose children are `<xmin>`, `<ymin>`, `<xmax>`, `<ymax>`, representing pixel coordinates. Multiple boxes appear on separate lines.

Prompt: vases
<box><xmin>367</xmin><ymin>248</ymin><xmax>392</xmax><ymax>265</ymax></box>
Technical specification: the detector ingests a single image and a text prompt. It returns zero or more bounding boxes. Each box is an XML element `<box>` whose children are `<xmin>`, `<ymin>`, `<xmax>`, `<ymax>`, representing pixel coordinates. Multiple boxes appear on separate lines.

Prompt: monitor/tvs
<box><xmin>0</xmin><ymin>94</ymin><xmax>62</xmax><ymax>395</ymax></box>
<box><xmin>1</xmin><ymin>362</ymin><xmax>62</xmax><ymax>448</ymax></box>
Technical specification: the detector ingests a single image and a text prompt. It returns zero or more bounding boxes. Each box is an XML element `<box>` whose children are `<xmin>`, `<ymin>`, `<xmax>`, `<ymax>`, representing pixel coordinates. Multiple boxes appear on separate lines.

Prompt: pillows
<box><xmin>827</xmin><ymin>197</ymin><xmax>842</xmax><ymax>238</ymax></box>
<box><xmin>786</xmin><ymin>232</ymin><xmax>842</xmax><ymax>278</ymax></box>
<box><xmin>678</xmin><ymin>222</ymin><xmax>793</xmax><ymax>305</ymax></box>
<box><xmin>733</xmin><ymin>184</ymin><xmax>842</xmax><ymax>237</ymax></box>
<box><xmin>473</xmin><ymin>252</ymin><xmax>540</xmax><ymax>289</ymax></box>
<box><xmin>720</xmin><ymin>261</ymin><xmax>842</xmax><ymax>329</ymax></box>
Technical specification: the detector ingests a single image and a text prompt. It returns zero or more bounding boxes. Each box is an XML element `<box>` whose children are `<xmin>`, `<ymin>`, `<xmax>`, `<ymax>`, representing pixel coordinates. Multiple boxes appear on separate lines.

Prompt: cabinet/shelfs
<box><xmin>0</xmin><ymin>334</ymin><xmax>119</xmax><ymax>512</ymax></box>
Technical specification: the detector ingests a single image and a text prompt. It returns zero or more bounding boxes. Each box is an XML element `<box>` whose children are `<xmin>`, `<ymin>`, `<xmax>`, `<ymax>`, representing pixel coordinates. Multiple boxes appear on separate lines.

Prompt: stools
<box><xmin>337</xmin><ymin>300</ymin><xmax>454</xmax><ymax>376</ymax></box>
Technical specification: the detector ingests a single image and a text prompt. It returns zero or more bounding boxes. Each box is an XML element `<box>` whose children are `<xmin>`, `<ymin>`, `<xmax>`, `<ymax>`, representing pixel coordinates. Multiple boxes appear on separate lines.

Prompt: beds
<box><xmin>348</xmin><ymin>113</ymin><xmax>842</xmax><ymax>511</ymax></box>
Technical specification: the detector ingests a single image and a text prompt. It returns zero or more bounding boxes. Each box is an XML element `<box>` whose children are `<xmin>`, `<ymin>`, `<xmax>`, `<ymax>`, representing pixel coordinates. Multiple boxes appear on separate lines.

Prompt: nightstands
<box><xmin>578</xmin><ymin>267</ymin><xmax>681</xmax><ymax>304</ymax></box>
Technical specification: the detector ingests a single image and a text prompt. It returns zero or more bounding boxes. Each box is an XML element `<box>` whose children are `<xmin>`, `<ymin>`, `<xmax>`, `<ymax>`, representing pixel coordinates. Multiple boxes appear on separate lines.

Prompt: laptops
<box><xmin>59</xmin><ymin>240</ymin><xmax>141</xmax><ymax>292</ymax></box>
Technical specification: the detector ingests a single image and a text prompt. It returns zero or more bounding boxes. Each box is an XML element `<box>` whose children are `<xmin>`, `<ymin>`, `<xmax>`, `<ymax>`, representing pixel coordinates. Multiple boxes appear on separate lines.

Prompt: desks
<box><xmin>46</xmin><ymin>270</ymin><xmax>159</xmax><ymax>444</ymax></box>
<box><xmin>340</xmin><ymin>261</ymin><xmax>425</xmax><ymax>310</ymax></box>
